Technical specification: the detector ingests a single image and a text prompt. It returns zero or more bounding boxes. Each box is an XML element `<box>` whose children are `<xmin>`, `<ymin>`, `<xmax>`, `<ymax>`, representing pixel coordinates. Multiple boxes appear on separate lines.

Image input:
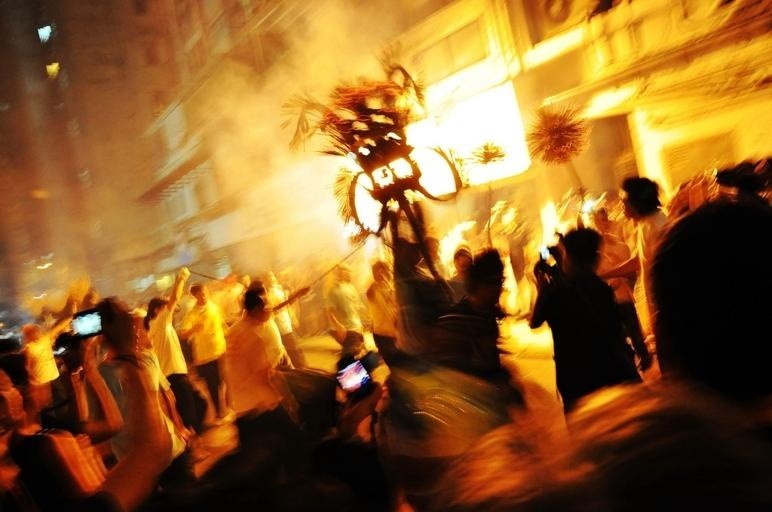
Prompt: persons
<box><xmin>0</xmin><ymin>159</ymin><xmax>771</xmax><ymax>511</ymax></box>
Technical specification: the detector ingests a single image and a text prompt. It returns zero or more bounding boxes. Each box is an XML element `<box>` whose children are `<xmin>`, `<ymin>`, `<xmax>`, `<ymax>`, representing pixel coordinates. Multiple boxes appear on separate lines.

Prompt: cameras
<box><xmin>73</xmin><ymin>308</ymin><xmax>102</xmax><ymax>340</ymax></box>
<box><xmin>541</xmin><ymin>248</ymin><xmax>559</xmax><ymax>270</ymax></box>
<box><xmin>334</xmin><ymin>354</ymin><xmax>377</xmax><ymax>397</ymax></box>
